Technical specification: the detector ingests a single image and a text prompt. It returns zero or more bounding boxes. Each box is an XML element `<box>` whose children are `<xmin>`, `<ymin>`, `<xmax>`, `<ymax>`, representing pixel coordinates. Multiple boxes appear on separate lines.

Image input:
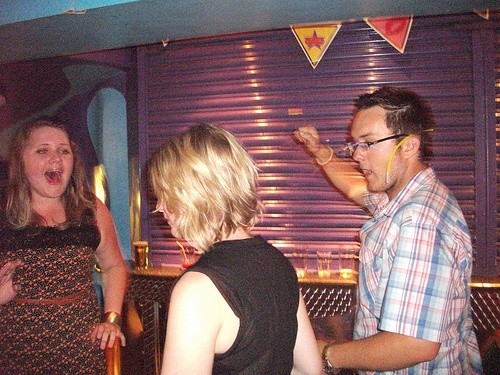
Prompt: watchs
<box><xmin>321</xmin><ymin>341</ymin><xmax>340</xmax><ymax>375</ymax></box>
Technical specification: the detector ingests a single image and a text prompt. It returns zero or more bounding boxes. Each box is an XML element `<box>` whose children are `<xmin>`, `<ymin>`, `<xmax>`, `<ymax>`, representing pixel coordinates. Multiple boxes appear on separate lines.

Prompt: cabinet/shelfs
<box><xmin>121</xmin><ymin>261</ymin><xmax>500</xmax><ymax>375</ymax></box>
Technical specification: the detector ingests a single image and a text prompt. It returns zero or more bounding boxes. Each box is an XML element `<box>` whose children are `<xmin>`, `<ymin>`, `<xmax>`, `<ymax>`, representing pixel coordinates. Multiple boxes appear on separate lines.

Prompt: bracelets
<box><xmin>100</xmin><ymin>310</ymin><xmax>125</xmax><ymax>327</ymax></box>
<box><xmin>315</xmin><ymin>146</ymin><xmax>334</xmax><ymax>166</ymax></box>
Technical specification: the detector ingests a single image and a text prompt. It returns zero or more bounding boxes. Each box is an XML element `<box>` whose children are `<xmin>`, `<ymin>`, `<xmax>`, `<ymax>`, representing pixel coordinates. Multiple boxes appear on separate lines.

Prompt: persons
<box><xmin>294</xmin><ymin>86</ymin><xmax>484</xmax><ymax>375</ymax></box>
<box><xmin>146</xmin><ymin>122</ymin><xmax>324</xmax><ymax>375</ymax></box>
<box><xmin>0</xmin><ymin>116</ymin><xmax>129</xmax><ymax>375</ymax></box>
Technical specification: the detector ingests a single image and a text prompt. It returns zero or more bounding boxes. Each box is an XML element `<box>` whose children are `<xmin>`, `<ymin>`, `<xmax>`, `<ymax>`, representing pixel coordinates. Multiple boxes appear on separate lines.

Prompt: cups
<box><xmin>317</xmin><ymin>251</ymin><xmax>331</xmax><ymax>278</ymax></box>
<box><xmin>338</xmin><ymin>248</ymin><xmax>355</xmax><ymax>280</ymax></box>
<box><xmin>180</xmin><ymin>247</ymin><xmax>195</xmax><ymax>270</ymax></box>
<box><xmin>292</xmin><ymin>249</ymin><xmax>308</xmax><ymax>279</ymax></box>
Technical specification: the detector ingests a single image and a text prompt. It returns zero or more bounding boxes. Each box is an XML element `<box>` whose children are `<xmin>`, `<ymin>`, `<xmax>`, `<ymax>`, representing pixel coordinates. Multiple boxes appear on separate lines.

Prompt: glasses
<box><xmin>336</xmin><ymin>132</ymin><xmax>408</xmax><ymax>157</ymax></box>
